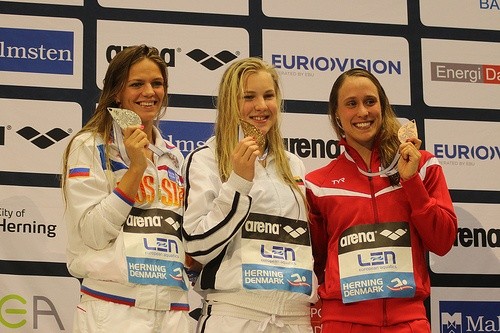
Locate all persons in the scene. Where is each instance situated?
[181,57,319,333]
[60,45,201,333]
[304,68,458,332]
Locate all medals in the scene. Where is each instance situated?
[107,107,142,130]
[398,119,418,145]
[239,118,265,149]
